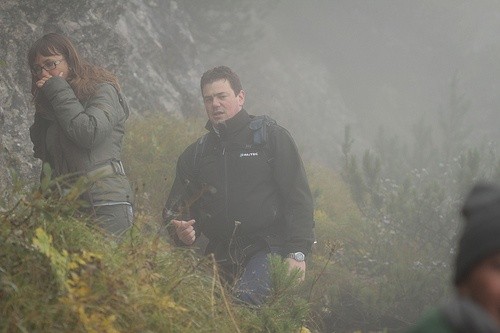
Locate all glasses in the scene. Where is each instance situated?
[31,59,66,76]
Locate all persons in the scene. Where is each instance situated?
[28,34,134,266]
[415,180,500,333]
[162,67,315,308]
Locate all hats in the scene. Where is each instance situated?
[454,182,500,285]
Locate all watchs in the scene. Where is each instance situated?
[287,252,305,262]
